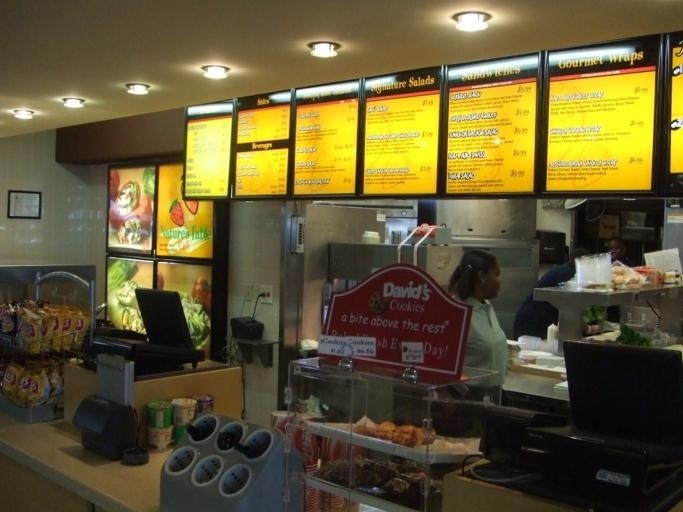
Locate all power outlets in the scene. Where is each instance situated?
[257,285,272,306]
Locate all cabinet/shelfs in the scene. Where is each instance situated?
[303,417,481,512]
[1,295,90,427]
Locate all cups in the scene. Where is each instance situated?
[271,410,364,512]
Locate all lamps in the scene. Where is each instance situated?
[307,41,341,58]
[125,83,150,96]
[14,110,34,120]
[201,65,230,80]
[62,98,85,109]
[451,11,492,33]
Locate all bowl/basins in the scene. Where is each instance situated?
[145,393,218,452]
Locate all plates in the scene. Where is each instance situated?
[621,305,659,330]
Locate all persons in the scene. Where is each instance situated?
[432,249,508,439]
[608,237,627,265]
[513,248,592,342]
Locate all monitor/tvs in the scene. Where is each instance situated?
[562,340,683,441]
[135,288,193,347]
